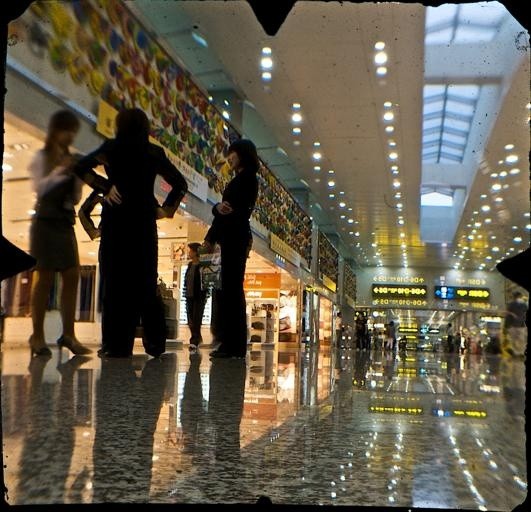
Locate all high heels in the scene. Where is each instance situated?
[56,333,94,357]
[29,334,53,357]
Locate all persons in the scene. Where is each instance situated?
[13,355,92,506]
[355,311,368,351]
[203,138,259,358]
[25,109,93,357]
[183,241,209,351]
[331,349,484,389]
[501,291,528,357]
[73,108,189,359]
[335,312,345,349]
[84,358,178,502]
[178,352,207,454]
[370,321,483,353]
[77,188,113,355]
[503,355,527,431]
[194,358,252,504]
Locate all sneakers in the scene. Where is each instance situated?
[188,343,201,349]
[96,342,168,358]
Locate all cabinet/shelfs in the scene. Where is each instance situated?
[245,298,279,344]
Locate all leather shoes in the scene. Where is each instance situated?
[209,348,247,359]
